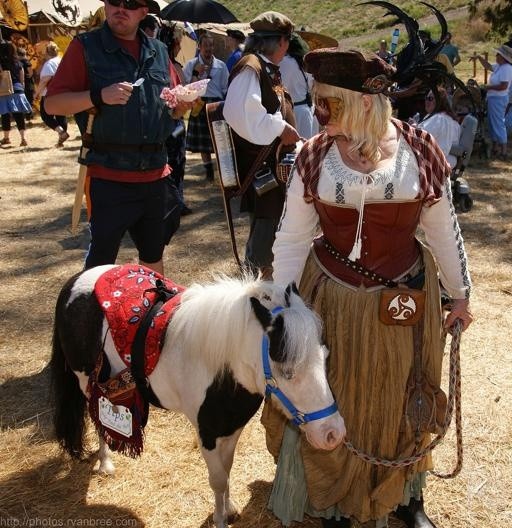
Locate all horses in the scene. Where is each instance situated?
[47,257,347,528]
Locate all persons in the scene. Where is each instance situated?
[33,43,69,146]
[222,10,301,282]
[136,16,327,194]
[267,43,473,527]
[375,32,509,162]
[44,2,194,278]
[2,42,34,146]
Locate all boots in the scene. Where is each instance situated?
[396,497,438,528]
[203,162,213,183]
[321,516,350,528]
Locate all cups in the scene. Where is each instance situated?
[252,168,279,198]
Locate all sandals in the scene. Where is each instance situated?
[0,131,70,146]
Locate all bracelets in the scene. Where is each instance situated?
[192,69,199,77]
[90,88,104,107]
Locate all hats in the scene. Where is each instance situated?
[302,48,397,94]
[493,45,512,64]
[249,11,293,37]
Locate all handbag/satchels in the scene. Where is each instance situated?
[403,385,447,435]
[0,66,15,96]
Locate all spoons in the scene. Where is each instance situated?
[132,76,145,87]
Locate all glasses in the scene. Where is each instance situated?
[109,0,147,10]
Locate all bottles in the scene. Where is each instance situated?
[412,112,419,122]
